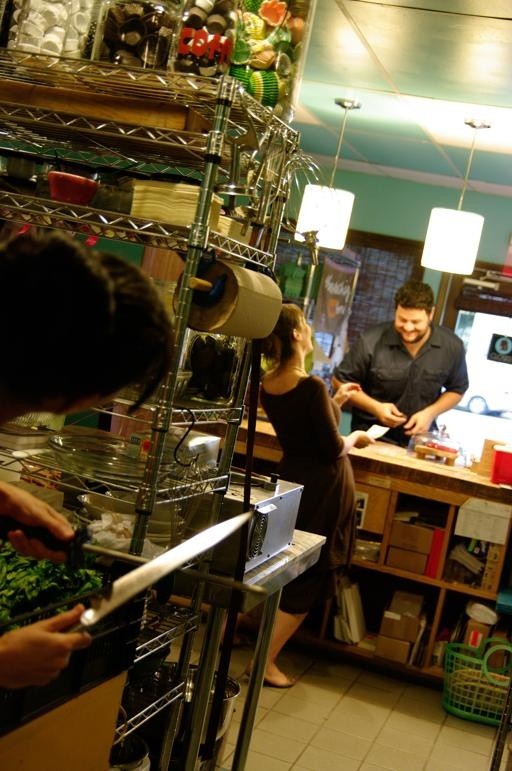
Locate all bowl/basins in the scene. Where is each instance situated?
[45,171,98,209]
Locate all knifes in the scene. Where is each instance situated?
[52,512,255,644]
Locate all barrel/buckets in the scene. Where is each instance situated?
[155,660,242,771]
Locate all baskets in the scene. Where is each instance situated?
[441,638,511,727]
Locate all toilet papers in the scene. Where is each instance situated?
[172,259,284,340]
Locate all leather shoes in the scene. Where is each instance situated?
[245,662,297,688]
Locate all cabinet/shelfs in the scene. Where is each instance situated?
[3,46,303,771]
[303,471,512,698]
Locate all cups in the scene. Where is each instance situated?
[476,439,512,486]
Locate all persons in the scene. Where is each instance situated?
[2,225,175,689]
[219,303,377,688]
[332,281,469,450]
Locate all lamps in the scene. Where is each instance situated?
[291,98,363,250]
[418,121,498,274]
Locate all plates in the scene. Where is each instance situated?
[72,490,185,543]
